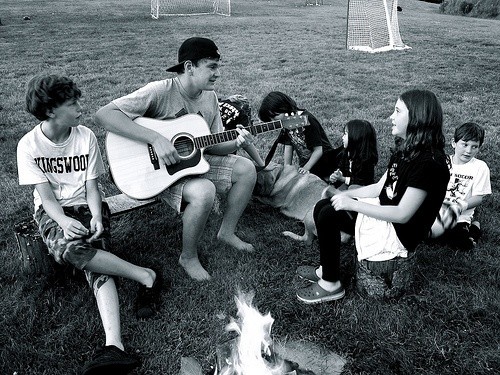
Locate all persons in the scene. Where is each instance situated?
[426,123,492,248]
[216,91,378,244]
[93,37,256,280]
[295,90,453,304]
[16,75,166,375]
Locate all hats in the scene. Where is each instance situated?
[166,36,223,73]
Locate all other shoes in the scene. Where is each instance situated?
[82,345,141,375]
[296,283,346,305]
[134,274,164,320]
[296,265,320,284]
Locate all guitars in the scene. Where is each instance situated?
[105,110,310,201]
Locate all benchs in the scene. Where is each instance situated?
[105,190,162,224]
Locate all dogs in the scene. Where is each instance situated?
[252,160,352,244]
